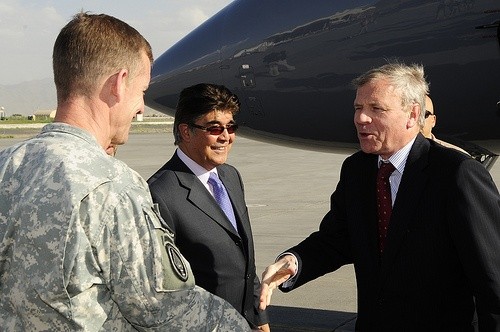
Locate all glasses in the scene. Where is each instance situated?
[425,110,433,119]
[185,121,238,135]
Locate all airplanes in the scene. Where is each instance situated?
[143,0,500,170]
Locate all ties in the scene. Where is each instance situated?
[208,172,238,234]
[377,160,396,255]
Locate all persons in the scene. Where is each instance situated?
[0,11,252,332]
[419,94,473,158]
[146,83,270,332]
[259,62,500,332]
